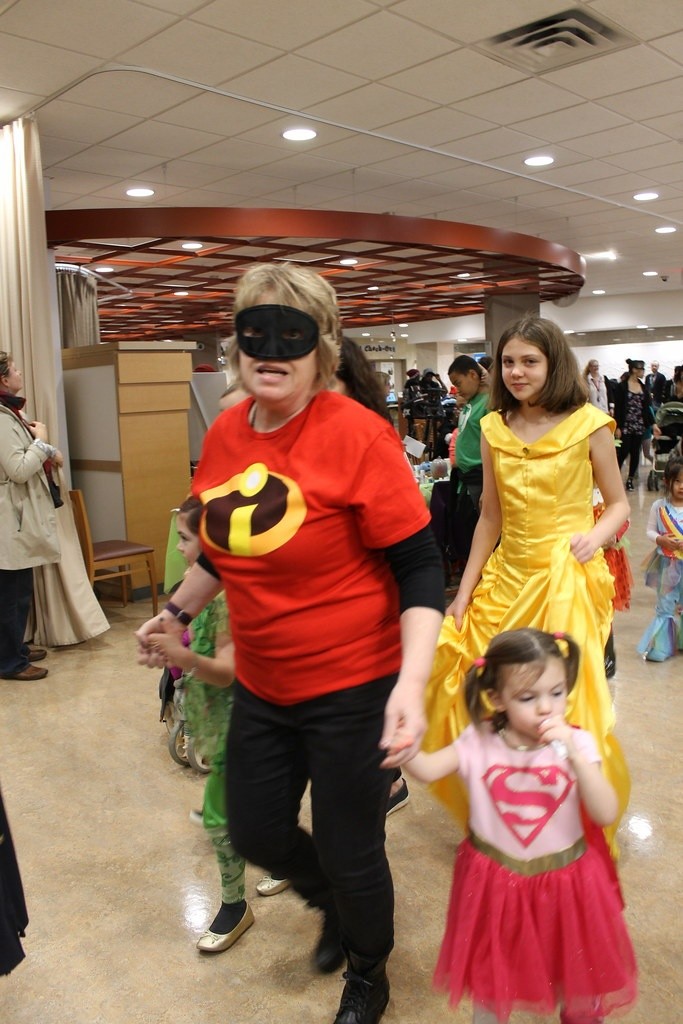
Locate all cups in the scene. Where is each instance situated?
[444,459,452,475]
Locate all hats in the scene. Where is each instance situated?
[407,369,419,379]
[421,368,437,377]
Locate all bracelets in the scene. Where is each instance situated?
[165,602,193,627]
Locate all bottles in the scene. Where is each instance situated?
[418,454,433,484]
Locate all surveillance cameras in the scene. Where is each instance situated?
[661,277,668,281]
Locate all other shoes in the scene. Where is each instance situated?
[316,910,346,973]
[333,960,390,1024]
[385,778,409,816]
[257,873,291,896]
[604,653,616,677]
[196,903,254,952]
[627,477,634,491]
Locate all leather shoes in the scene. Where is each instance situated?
[27,649,47,662]
[0,665,48,680]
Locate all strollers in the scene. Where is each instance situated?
[647,402,683,492]
[432,467,473,586]
[159,663,219,774]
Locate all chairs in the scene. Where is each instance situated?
[67,490,157,619]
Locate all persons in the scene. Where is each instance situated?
[592,476,631,679]
[582,358,614,416]
[641,361,683,464]
[402,369,448,405]
[0,350,62,681]
[388,627,641,1024]
[421,318,633,857]
[142,496,291,952]
[331,336,409,814]
[637,455,683,661]
[611,358,662,491]
[134,264,447,1024]
[447,354,494,515]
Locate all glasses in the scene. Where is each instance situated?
[637,367,645,370]
